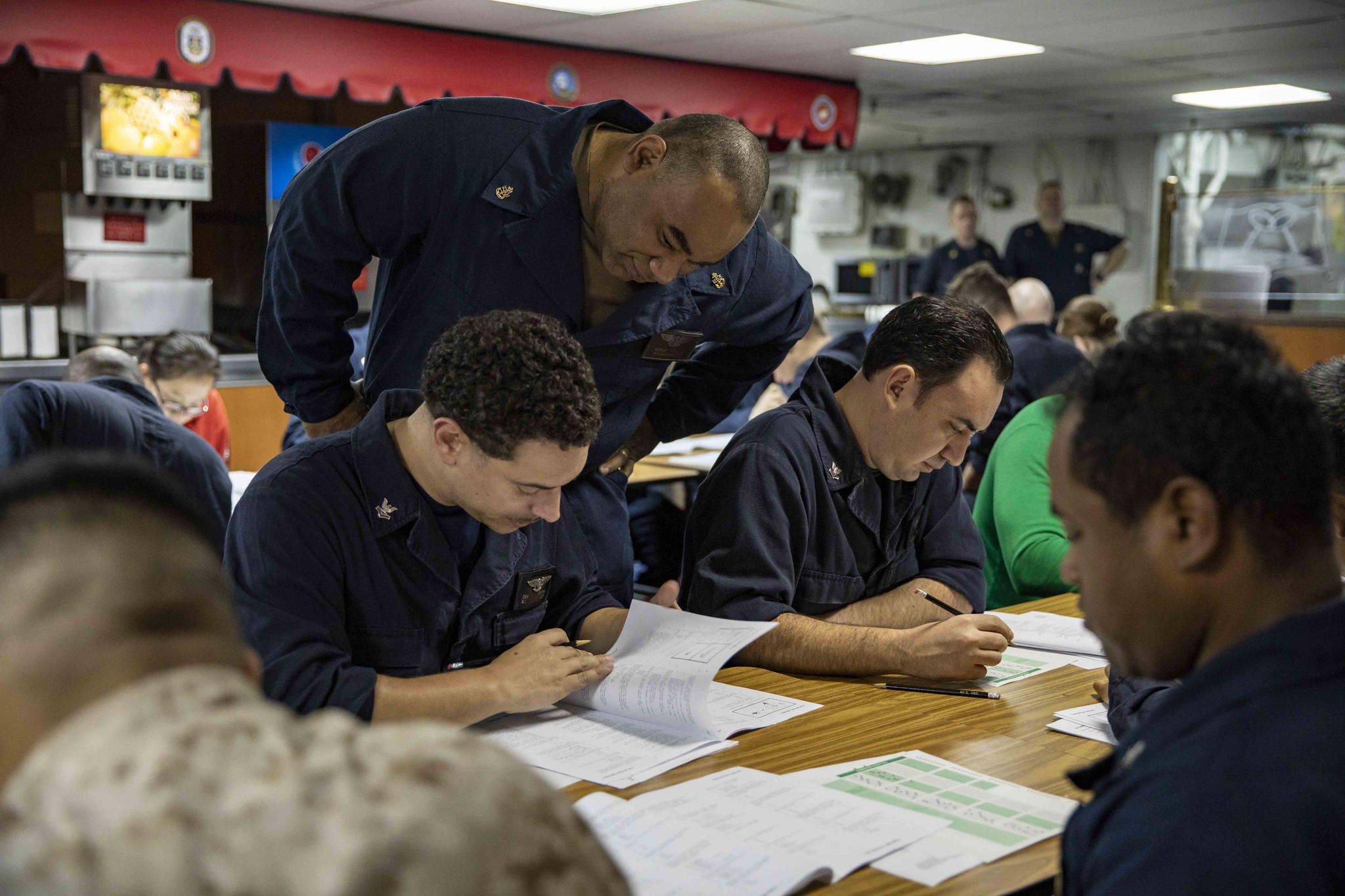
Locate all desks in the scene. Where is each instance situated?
[626,431,736,508]
[452,591,1119,896]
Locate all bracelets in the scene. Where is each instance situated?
[1096,273,1103,281]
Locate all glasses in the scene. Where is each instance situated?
[148,371,208,416]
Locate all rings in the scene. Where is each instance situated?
[621,450,628,458]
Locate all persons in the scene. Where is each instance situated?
[623,264,1121,612]
[1004,178,1127,314]
[0,447,629,896]
[255,99,814,501]
[281,309,371,453]
[911,194,1003,299]
[676,293,1013,682]
[222,311,636,738]
[0,331,234,530]
[1040,306,1345,894]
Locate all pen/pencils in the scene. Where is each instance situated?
[448,640,594,668]
[914,588,1016,648]
[871,683,1001,699]
